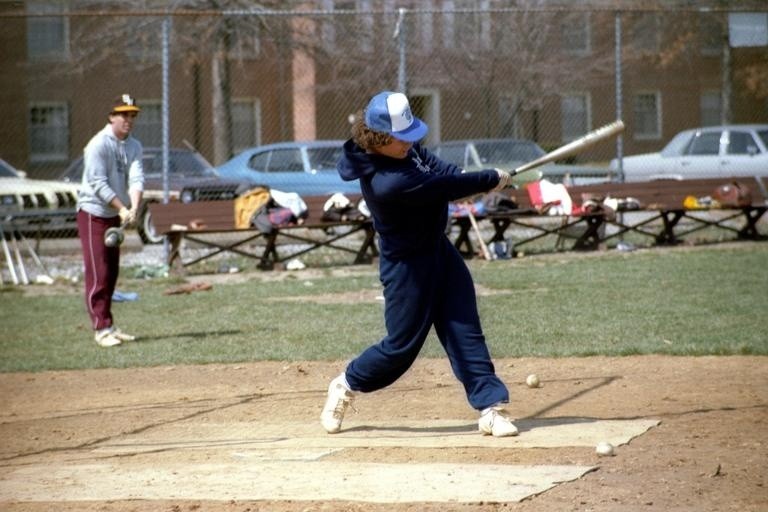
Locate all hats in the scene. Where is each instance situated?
[108,93,143,116]
[363,91,429,143]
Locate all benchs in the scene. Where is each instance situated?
[145,176,767,277]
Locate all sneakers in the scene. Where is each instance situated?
[322,373,356,435]
[476,405,520,439]
[93,323,136,349]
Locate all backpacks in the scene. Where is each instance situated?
[230,183,308,231]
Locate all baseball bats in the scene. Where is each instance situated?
[104,220,128,248]
[507,119,626,177]
[464,201,492,261]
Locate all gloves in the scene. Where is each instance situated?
[118,208,134,224]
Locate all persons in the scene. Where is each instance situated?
[319,91,519,437]
[76,94,145,347]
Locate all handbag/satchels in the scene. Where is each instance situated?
[481,193,519,215]
[603,197,642,213]
[581,198,604,214]
[684,195,720,209]
[714,180,751,209]
[524,180,580,216]
[448,200,486,218]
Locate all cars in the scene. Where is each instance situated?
[0,158,81,234]
[425,138,622,187]
[608,124,768,183]
[58,146,254,245]
[213,140,364,195]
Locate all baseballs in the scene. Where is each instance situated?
[527,374,539,387]
[596,443,613,457]
